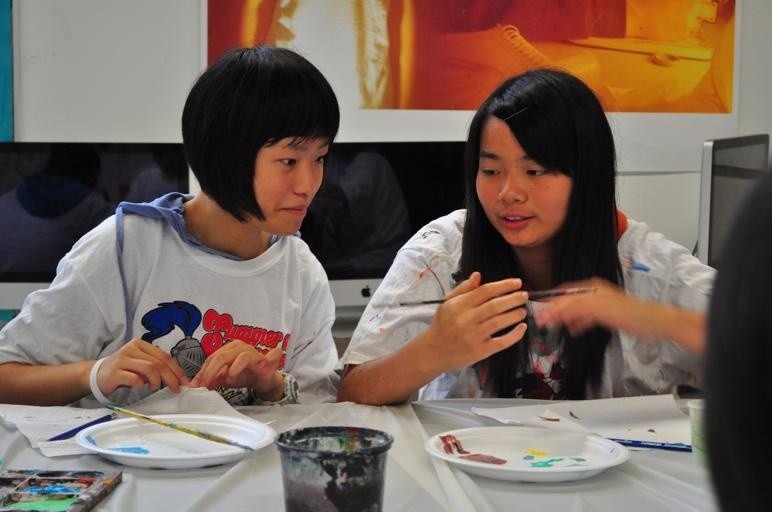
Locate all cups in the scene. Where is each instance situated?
[274,428,393,511]
[687,398,711,484]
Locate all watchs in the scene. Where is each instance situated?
[251,369,299,405]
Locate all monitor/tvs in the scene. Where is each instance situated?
[1,140,191,312]
[299,139,475,311]
[697,131,772,271]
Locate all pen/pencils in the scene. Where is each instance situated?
[46,412,118,442]
[608,438,692,453]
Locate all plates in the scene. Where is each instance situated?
[425,425,632,484]
[77,414,278,467]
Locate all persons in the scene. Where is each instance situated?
[337,66,719,406]
[0,43,341,408]
[0,141,114,286]
[310,129,416,283]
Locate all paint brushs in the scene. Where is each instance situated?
[371,286,599,309]
[102,403,255,452]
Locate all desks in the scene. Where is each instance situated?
[0,395,720,512]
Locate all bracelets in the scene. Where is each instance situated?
[87,354,132,407]
